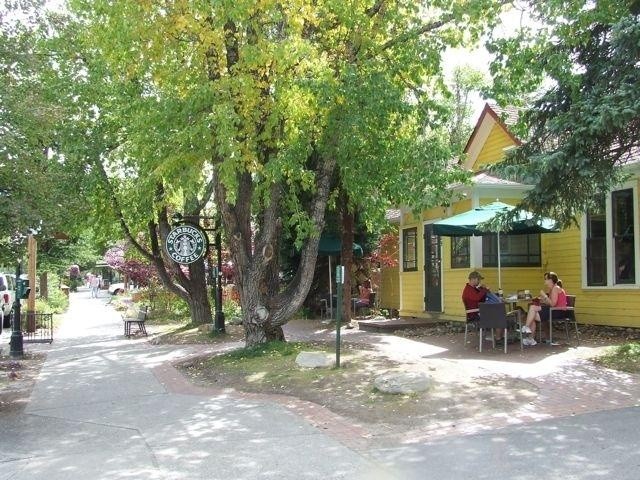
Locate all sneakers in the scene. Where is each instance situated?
[494,325,537,350]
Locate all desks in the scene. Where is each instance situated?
[485,293,540,313]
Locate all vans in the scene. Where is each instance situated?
[0,269,31,337]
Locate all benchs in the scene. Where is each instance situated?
[119,304,151,338]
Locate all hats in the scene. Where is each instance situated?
[469,271,485,278]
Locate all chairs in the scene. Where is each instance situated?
[477,302,525,353]
[538,294,582,347]
[321,290,377,322]
[463,297,498,349]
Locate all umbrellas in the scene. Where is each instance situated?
[434,196,562,297]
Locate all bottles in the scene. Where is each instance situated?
[498,288,503,299]
[524,289,531,300]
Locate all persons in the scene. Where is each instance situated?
[516,271,568,348]
[84,270,105,297]
[294,232,364,295]
[462,270,507,346]
[353,280,372,314]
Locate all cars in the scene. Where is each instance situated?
[108,280,138,295]
